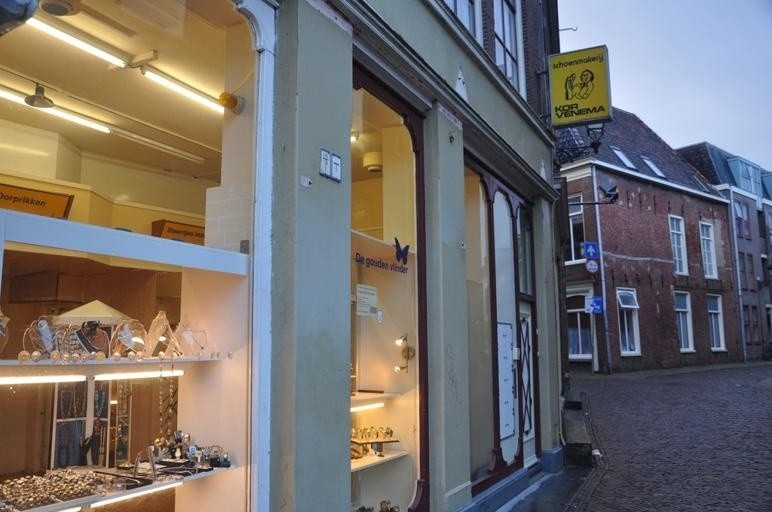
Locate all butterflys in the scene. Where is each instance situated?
[394,236,410,266]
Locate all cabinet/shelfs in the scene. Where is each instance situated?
[0,355,233,512]
[350,391,408,473]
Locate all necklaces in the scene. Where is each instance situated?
[163,466,200,477]
[82,321,223,352]
[117,475,142,487]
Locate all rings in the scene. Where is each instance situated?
[0,473,99,509]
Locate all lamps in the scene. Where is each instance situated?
[568,184,619,206]
[552,123,603,172]
[394,332,415,374]
[24,86,54,111]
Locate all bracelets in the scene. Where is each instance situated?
[348,421,396,460]
